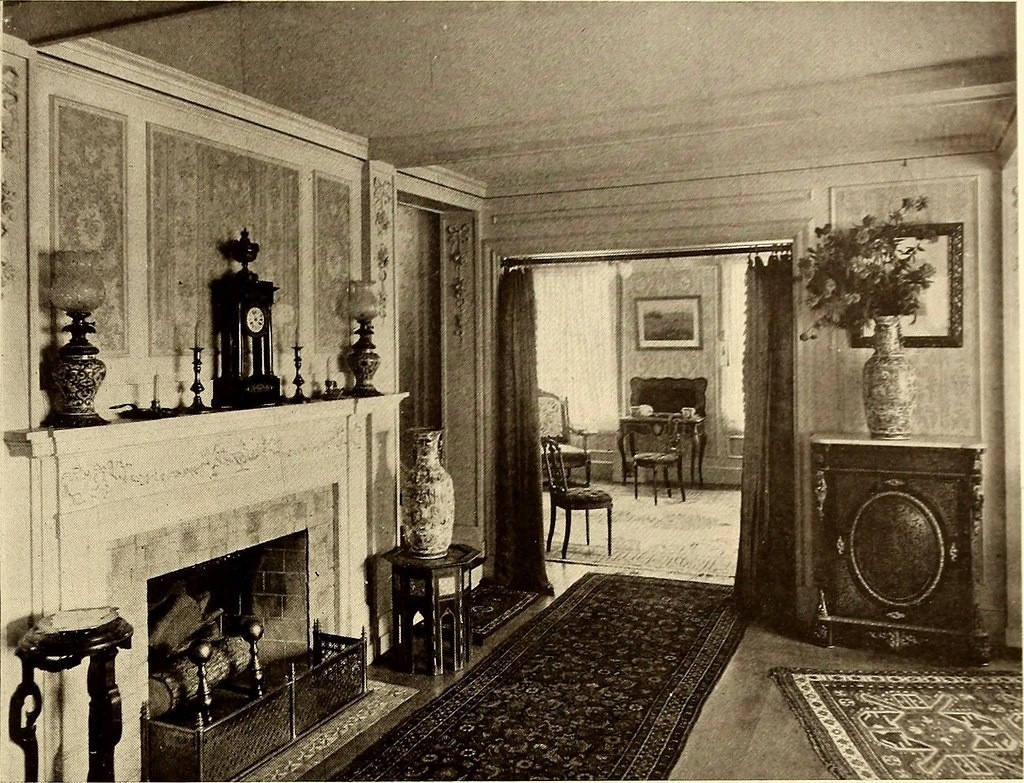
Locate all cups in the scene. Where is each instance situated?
[681,408,695,419]
[631,407,641,416]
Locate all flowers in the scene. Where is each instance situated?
[798,195,938,341]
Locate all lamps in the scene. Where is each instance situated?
[348,280,380,349]
[48,251,107,356]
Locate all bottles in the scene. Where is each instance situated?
[402,424,456,560]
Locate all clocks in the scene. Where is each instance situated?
[212,227,283,409]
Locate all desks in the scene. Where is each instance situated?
[617,412,708,489]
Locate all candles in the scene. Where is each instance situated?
[295,328,299,346]
[153,370,160,401]
[195,320,204,347]
[327,358,331,381]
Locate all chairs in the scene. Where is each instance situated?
[633,413,686,506]
[540,436,613,559]
[538,388,597,487]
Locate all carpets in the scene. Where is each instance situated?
[768,666,1023,781]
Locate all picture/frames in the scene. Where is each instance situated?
[633,295,704,351]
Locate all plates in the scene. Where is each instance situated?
[654,412,681,417]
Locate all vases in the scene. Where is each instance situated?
[347,347,384,398]
[42,354,111,427]
[402,428,455,560]
[862,316,918,441]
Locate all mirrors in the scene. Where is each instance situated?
[850,222,964,349]
